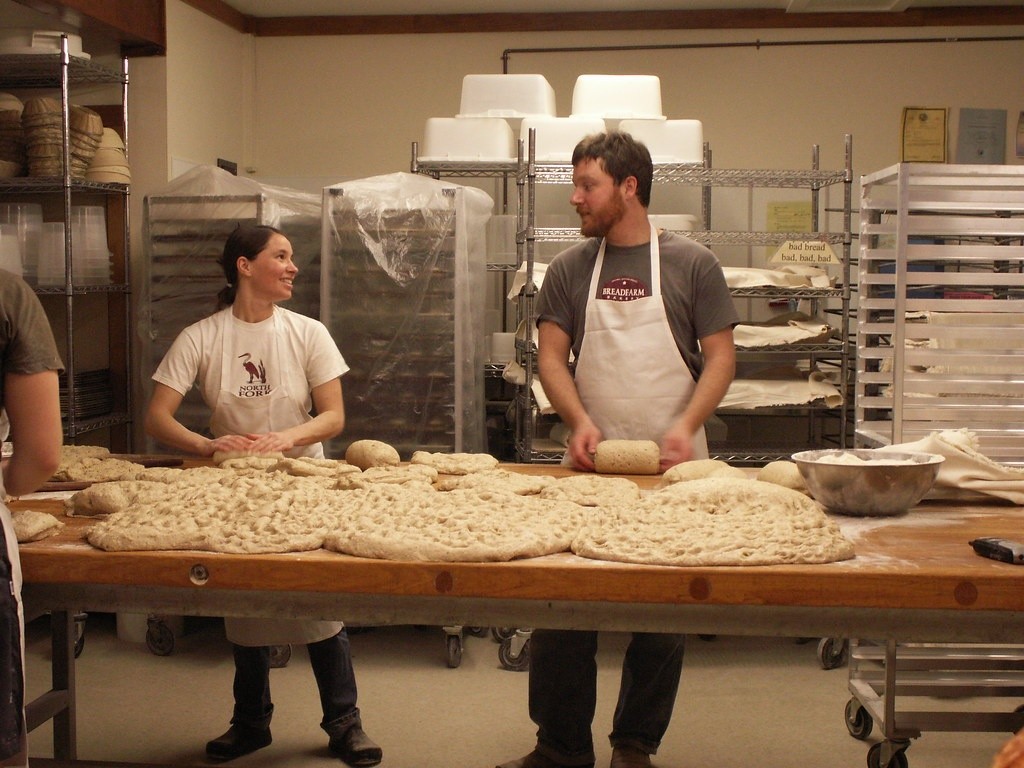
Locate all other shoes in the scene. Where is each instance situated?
[327,726,382,766]
[206,724,272,762]
[608,744,650,768]
[495,748,561,767]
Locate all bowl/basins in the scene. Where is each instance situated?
[790,448,946,515]
[86,127,131,186]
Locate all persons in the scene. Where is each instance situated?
[0,262,68,768]
[490,131,740,768]
[148,223,386,768]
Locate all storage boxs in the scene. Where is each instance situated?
[648,214,703,232]
[570,74,668,120]
[516,118,609,161]
[455,74,557,118]
[492,333,517,362]
[0,202,115,287]
[618,119,704,163]
[0,28,92,60]
[417,117,518,162]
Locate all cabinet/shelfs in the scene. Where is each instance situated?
[843,161,1023,767]
[140,186,488,669]
[0,35,135,659]
[409,129,1023,669]
